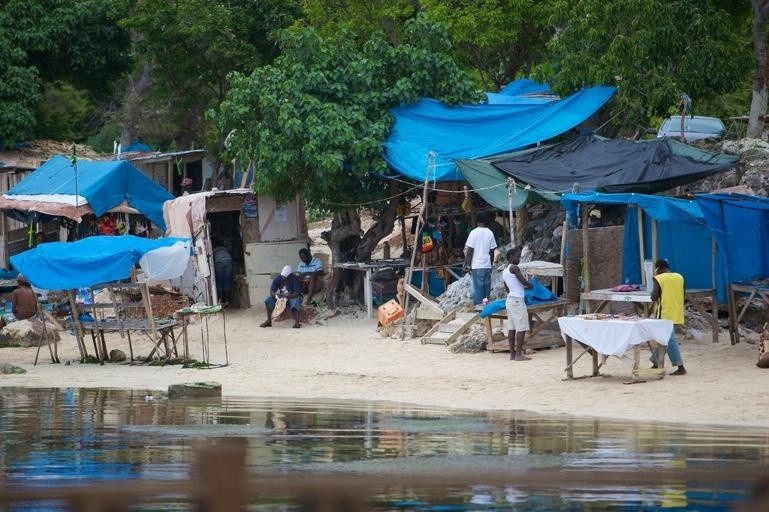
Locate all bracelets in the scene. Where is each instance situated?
[467,266,470,269]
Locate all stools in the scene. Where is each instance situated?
[180,302,230,371]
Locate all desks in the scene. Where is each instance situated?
[517,260,566,297]
[481,296,571,354]
[557,313,675,383]
[579,286,720,344]
[727,280,768,346]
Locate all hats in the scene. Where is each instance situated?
[281,266,292,278]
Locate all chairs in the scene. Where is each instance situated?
[307,251,334,306]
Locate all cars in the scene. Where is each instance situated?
[655,115,727,144]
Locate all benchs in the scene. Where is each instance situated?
[66,282,184,362]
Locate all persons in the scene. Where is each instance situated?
[259,264,305,330]
[294,247,325,306]
[212,243,235,306]
[38,231,46,242]
[461,210,497,308]
[11,273,38,320]
[180,177,193,196]
[501,249,534,361]
[647,258,687,376]
